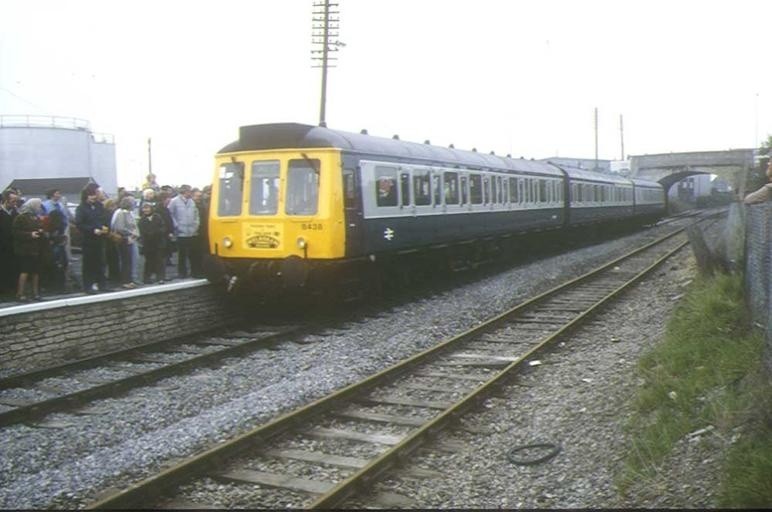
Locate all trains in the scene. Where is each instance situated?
[201,121,667,302]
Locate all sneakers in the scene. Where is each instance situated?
[14,294,44,303]
[121,282,136,289]
[85,286,113,295]
[158,278,171,284]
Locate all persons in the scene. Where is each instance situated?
[0,173,212,303]
[744,157,772,205]
